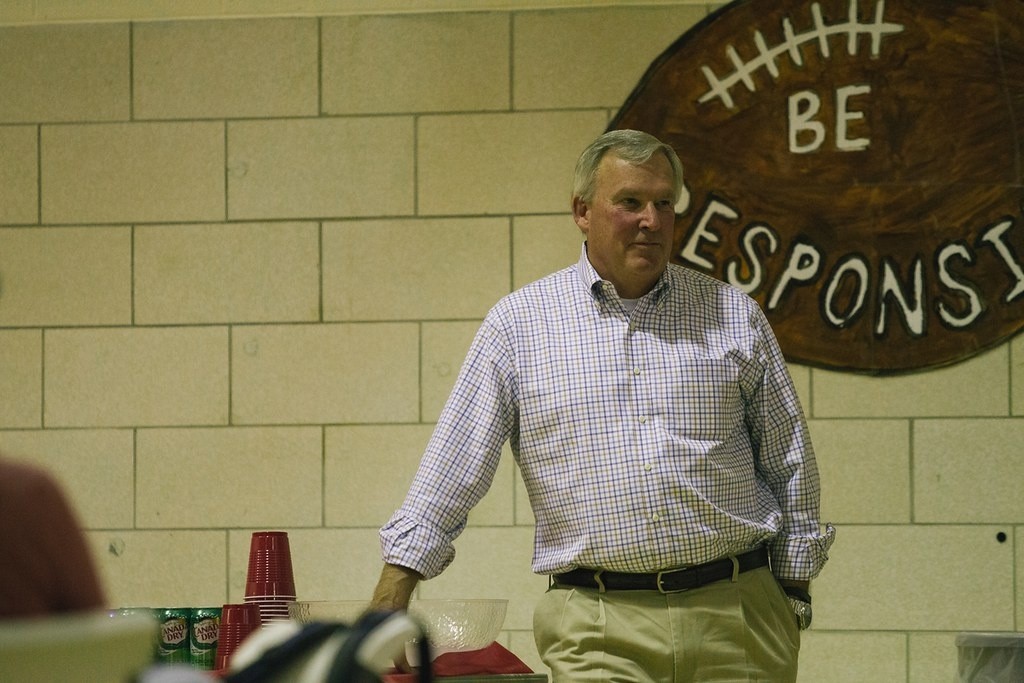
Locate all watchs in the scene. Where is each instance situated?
[789,598,813,631]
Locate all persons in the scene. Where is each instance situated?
[0,457,157,683]
[362,128,837,683]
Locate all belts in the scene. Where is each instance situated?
[552,547,768,594]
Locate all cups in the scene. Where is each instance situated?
[212,530,297,673]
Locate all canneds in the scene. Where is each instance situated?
[110,607,222,672]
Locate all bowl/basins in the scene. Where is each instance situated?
[287,598,511,675]
